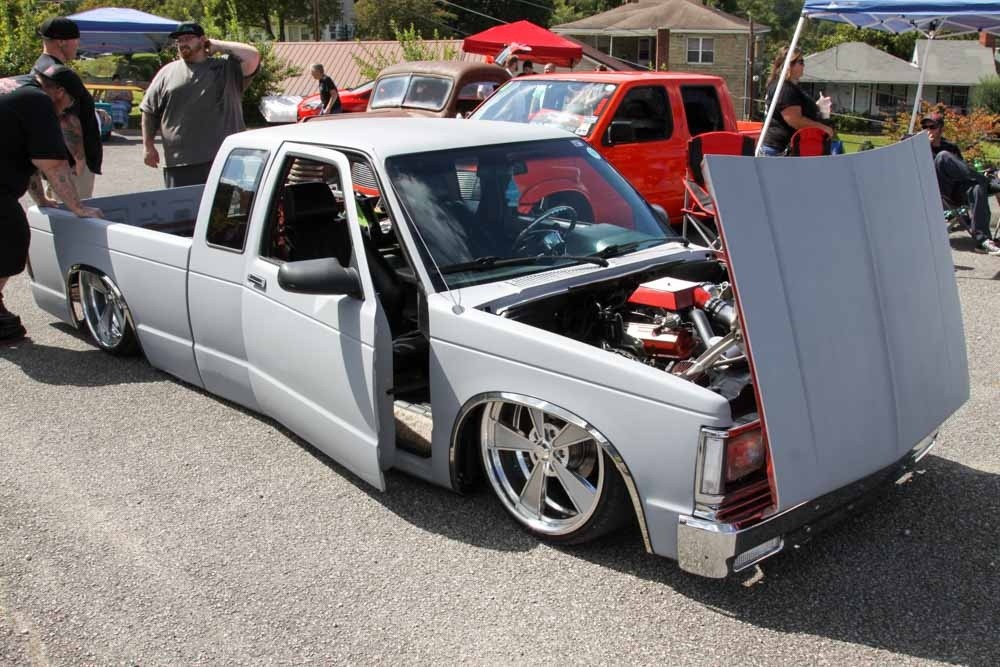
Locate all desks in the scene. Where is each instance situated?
[83,83,144,103]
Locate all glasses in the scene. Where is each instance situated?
[922,125,938,130]
[795,59,806,64]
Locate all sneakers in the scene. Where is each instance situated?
[975,239,1000,255]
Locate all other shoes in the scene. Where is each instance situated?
[1,294,27,340]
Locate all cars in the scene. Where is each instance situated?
[295,76,478,122]
[287,57,518,188]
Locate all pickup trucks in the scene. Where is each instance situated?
[20,107,974,590]
[352,70,765,228]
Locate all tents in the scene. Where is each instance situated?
[463,20,583,68]
[65,7,182,66]
[754,0,1000,158]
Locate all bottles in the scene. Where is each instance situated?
[974,159,993,171]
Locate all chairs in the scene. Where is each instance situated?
[680,131,756,250]
[785,127,832,157]
[285,182,430,389]
[903,132,973,235]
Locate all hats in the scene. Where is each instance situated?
[33,64,85,103]
[920,112,944,125]
[40,17,81,41]
[168,23,205,36]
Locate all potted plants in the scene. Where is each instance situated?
[242,36,306,124]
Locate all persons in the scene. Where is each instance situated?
[139,23,261,188]
[592,64,606,71]
[544,63,555,73]
[29,18,103,204]
[503,54,518,77]
[517,60,538,77]
[0,65,104,340]
[311,64,342,115]
[901,114,1000,256]
[759,46,834,156]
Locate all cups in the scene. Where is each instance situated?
[816,97,831,119]
